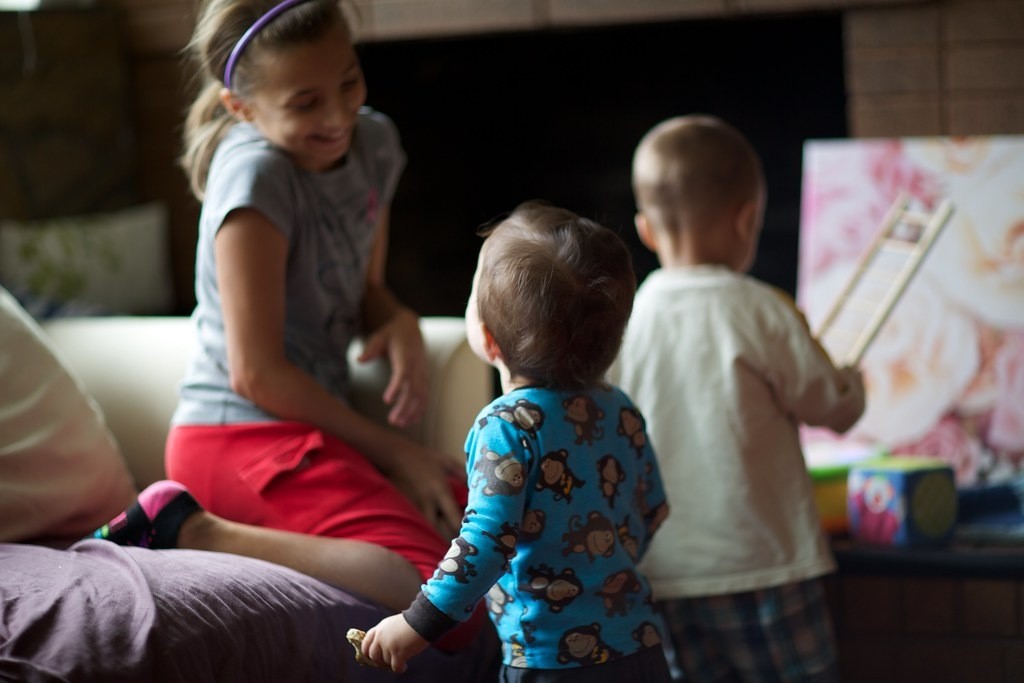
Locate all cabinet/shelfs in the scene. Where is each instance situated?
[831,534,1024,682]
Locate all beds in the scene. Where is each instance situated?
[0,311,491,683]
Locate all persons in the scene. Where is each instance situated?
[79,0,470,613]
[360,201,676,683]
[600,116,867,683]
